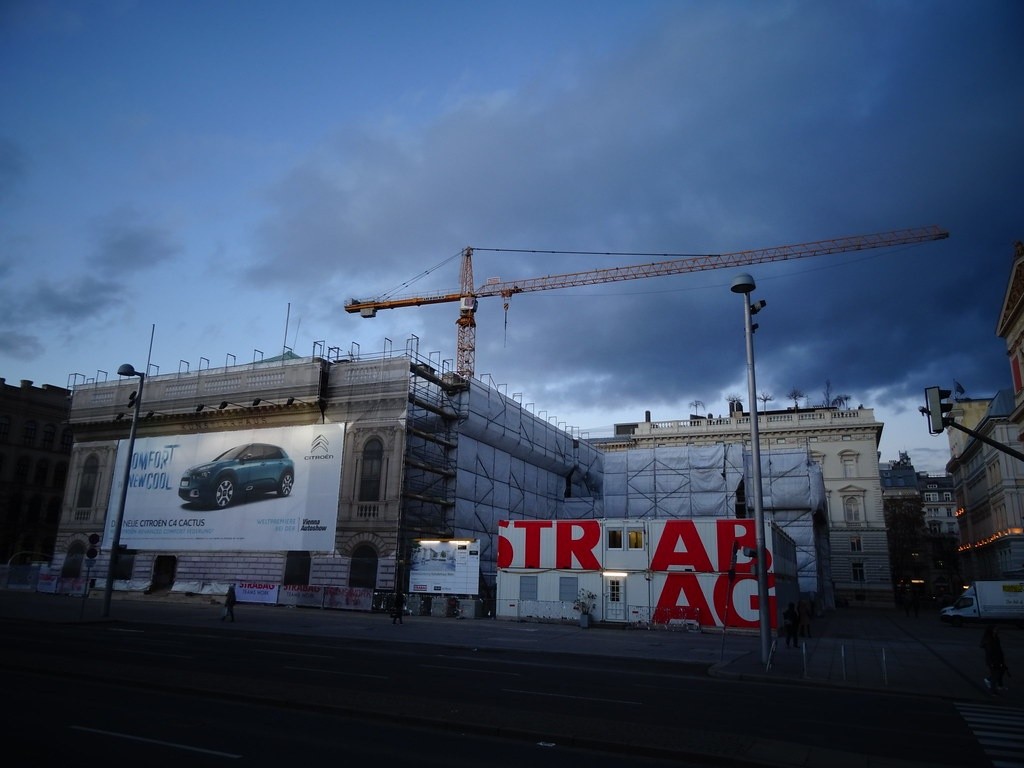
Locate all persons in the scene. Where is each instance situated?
[782,602,800,649]
[902,589,920,617]
[392,588,406,625]
[797,598,812,638]
[981,624,1008,691]
[221,586,236,623]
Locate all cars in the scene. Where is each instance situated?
[178,443,295,509]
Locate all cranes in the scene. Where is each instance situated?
[344,224,950,379]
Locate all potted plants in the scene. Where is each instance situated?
[573,588,597,629]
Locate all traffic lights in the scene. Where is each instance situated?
[938,387,957,429]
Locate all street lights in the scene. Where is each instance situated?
[724,267,776,675]
[100,362,147,621]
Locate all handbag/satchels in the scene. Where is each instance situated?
[221,607,228,616]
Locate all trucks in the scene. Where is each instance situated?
[940,579,1024,627]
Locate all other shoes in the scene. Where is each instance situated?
[984,678,991,689]
[997,686,1008,691]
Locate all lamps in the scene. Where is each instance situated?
[286,396,312,406]
[144,410,167,419]
[219,400,246,409]
[116,412,143,421]
[253,398,278,407]
[196,404,218,412]
